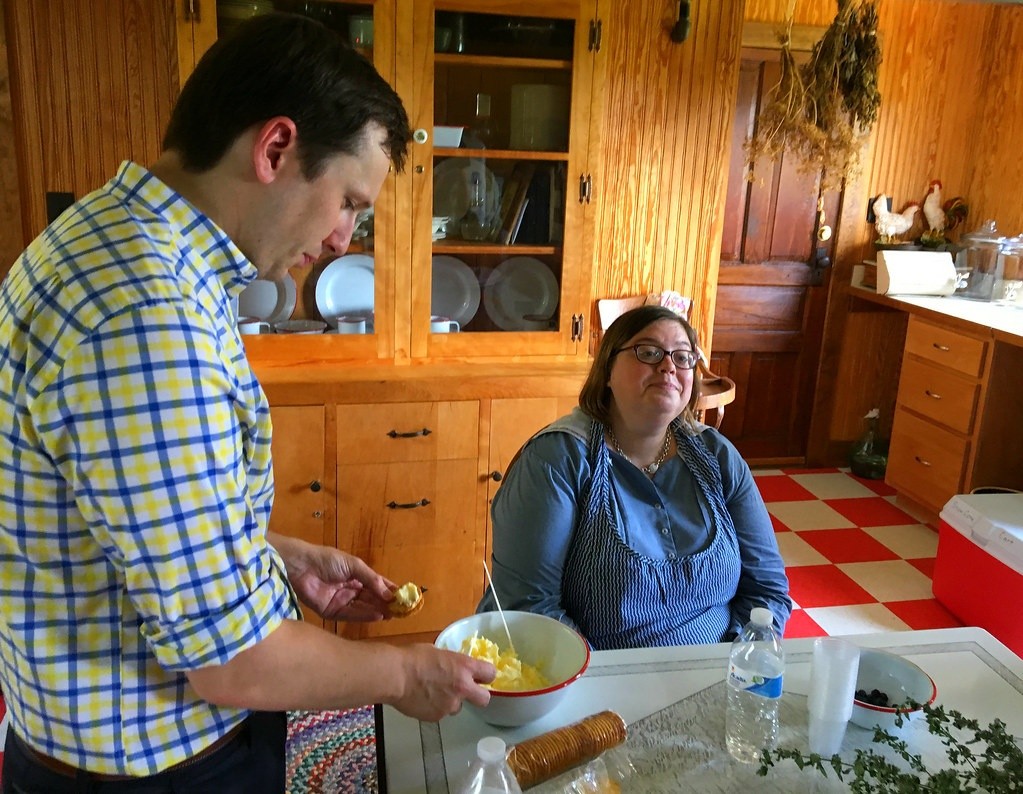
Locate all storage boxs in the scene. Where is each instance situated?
[932,492,1023,660]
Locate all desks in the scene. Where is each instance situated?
[374,626,1023,792]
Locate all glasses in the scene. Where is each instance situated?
[616,344,701,369]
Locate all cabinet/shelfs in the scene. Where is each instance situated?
[882,311,1023,531]
[175,0,596,647]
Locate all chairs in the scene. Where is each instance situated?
[599,294,737,432]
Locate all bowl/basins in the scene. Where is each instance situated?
[873,238,952,251]
[274,320,327,334]
[850,647,937,730]
[434,610,590,730]
[434,126,464,148]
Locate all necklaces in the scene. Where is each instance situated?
[605,422,673,475]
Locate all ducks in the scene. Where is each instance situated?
[848,407,888,480]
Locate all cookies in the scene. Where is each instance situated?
[506,710,627,790]
[388,585,424,619]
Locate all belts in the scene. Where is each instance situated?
[14,717,244,782]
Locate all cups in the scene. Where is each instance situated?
[238,316,271,334]
[452,115,500,149]
[430,315,460,333]
[431,217,450,241]
[808,637,860,759]
[298,1,336,30]
[349,15,374,48]
[509,84,568,152]
[435,28,452,51]
[336,317,365,332]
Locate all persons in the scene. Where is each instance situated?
[0,12,496,794]
[474,305,792,652]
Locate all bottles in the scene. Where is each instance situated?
[584,679,729,793]
[521,161,551,245]
[450,14,466,53]
[216,0,277,41]
[848,408,889,480]
[460,736,522,794]
[954,219,1023,302]
[726,609,786,764]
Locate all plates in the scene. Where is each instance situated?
[238,270,298,331]
[483,257,559,331]
[434,158,499,235]
[316,254,375,328]
[432,256,481,329]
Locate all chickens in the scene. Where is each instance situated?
[923,180,968,237]
[872,192,921,243]
